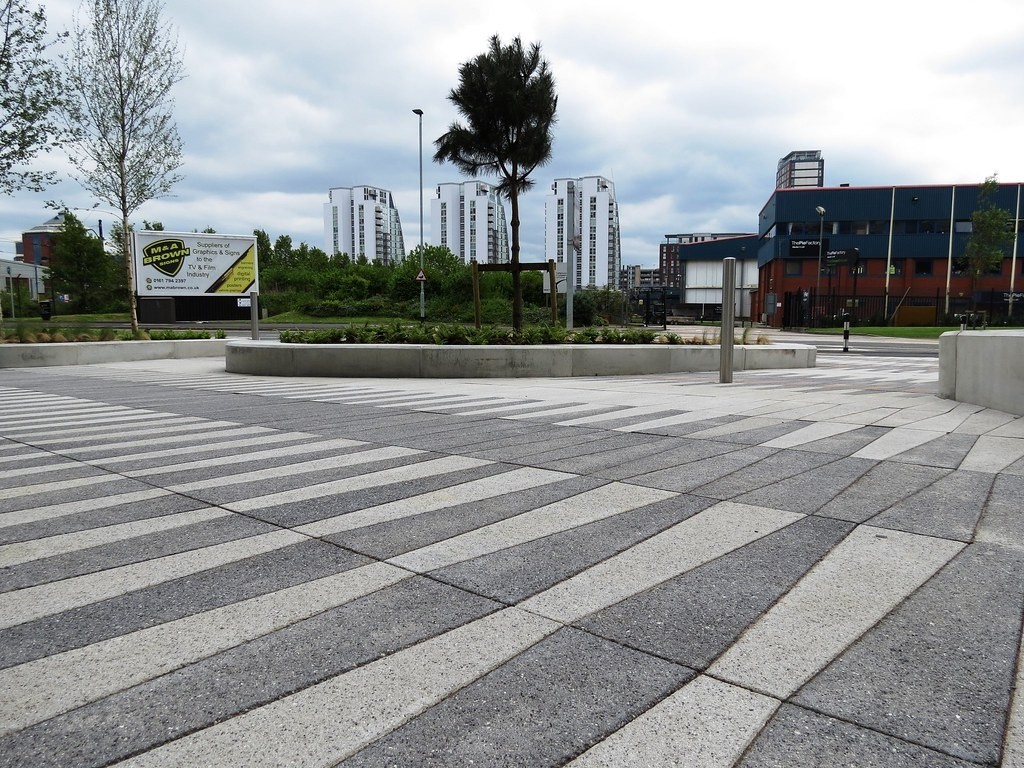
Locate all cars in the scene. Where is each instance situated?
[642,312,673,325]
[628,314,644,322]
[959,313,992,326]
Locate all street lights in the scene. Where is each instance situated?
[851,248,859,310]
[41,257,56,316]
[412,109,426,324]
[7,266,15,319]
[17,274,22,318]
[82,219,106,248]
[815,205,826,298]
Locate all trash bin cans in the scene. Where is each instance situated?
[39,302,52,320]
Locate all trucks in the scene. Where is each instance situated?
[657,312,695,325]
